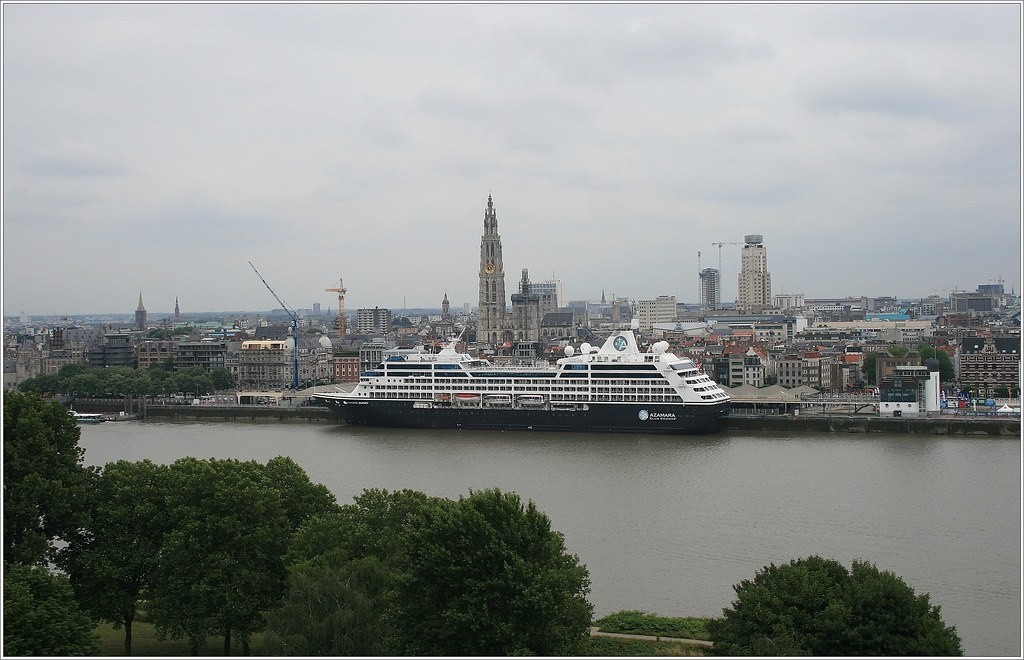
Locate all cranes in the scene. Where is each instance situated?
[713,242,741,275]
[324,278,349,346]
[248,261,300,392]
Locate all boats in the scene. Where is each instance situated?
[454,393,480,404]
[482,395,512,406]
[433,394,451,403]
[66,403,106,423]
[517,394,545,406]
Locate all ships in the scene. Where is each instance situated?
[311,327,732,436]
